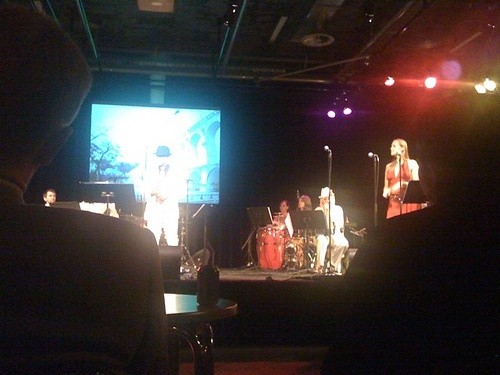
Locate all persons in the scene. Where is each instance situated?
[43,188,56,207]
[0,0,170,375]
[383,139,423,219]
[140,146,179,246]
[322,91,500,375]
[274,187,348,273]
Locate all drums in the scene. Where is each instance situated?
[257,229,286,269]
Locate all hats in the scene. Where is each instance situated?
[154,145,171,157]
[319,187,333,198]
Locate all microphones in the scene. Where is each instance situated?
[367,152,379,159]
[324,145,332,154]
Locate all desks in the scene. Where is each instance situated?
[164,293,242,375]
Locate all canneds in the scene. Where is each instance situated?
[196,264,220,305]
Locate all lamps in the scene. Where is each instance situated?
[328,83,354,119]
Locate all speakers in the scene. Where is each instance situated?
[159,246,183,279]
[191,248,209,269]
